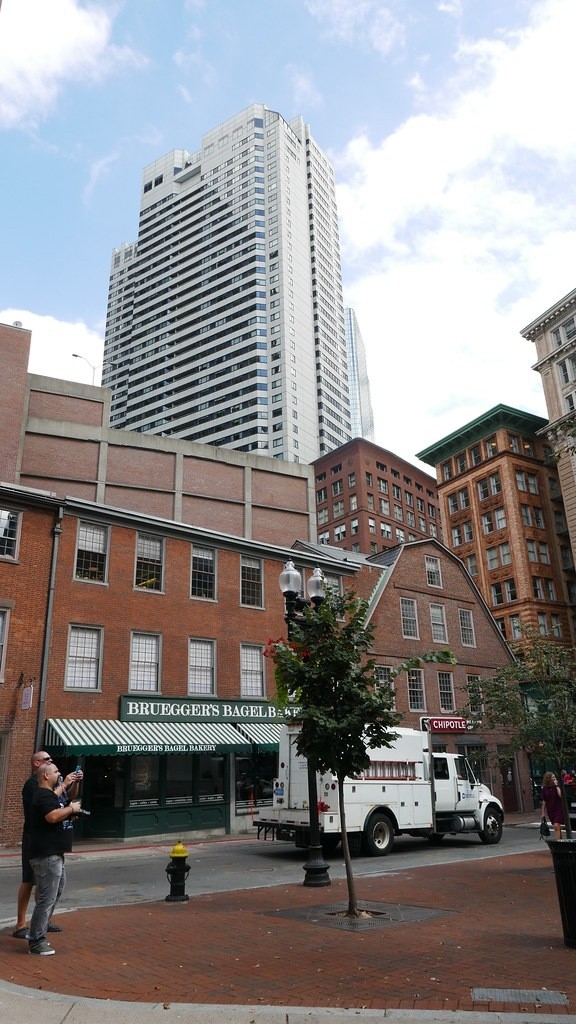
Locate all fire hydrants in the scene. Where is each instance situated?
[163,840,192,901]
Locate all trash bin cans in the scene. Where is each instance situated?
[543,838,576,950]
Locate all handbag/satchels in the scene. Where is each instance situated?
[539,823,551,836]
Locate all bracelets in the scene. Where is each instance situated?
[69,805,75,814]
[555,785,560,787]
[60,784,66,789]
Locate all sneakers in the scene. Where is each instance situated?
[30,935,56,956]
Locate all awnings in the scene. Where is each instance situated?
[235,722,283,752]
[43,718,253,760]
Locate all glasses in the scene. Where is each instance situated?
[38,756,53,761]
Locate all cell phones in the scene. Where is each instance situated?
[76,765,81,782]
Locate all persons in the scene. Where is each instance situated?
[9,750,83,941]
[539,772,568,840]
[560,770,576,784]
[235,772,265,799]
[24,762,82,957]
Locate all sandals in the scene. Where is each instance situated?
[47,922,62,933]
[13,925,30,938]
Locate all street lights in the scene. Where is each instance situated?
[278,555,333,887]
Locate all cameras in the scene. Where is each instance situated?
[72,799,90,816]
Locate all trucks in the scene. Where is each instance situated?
[252,719,505,858]
